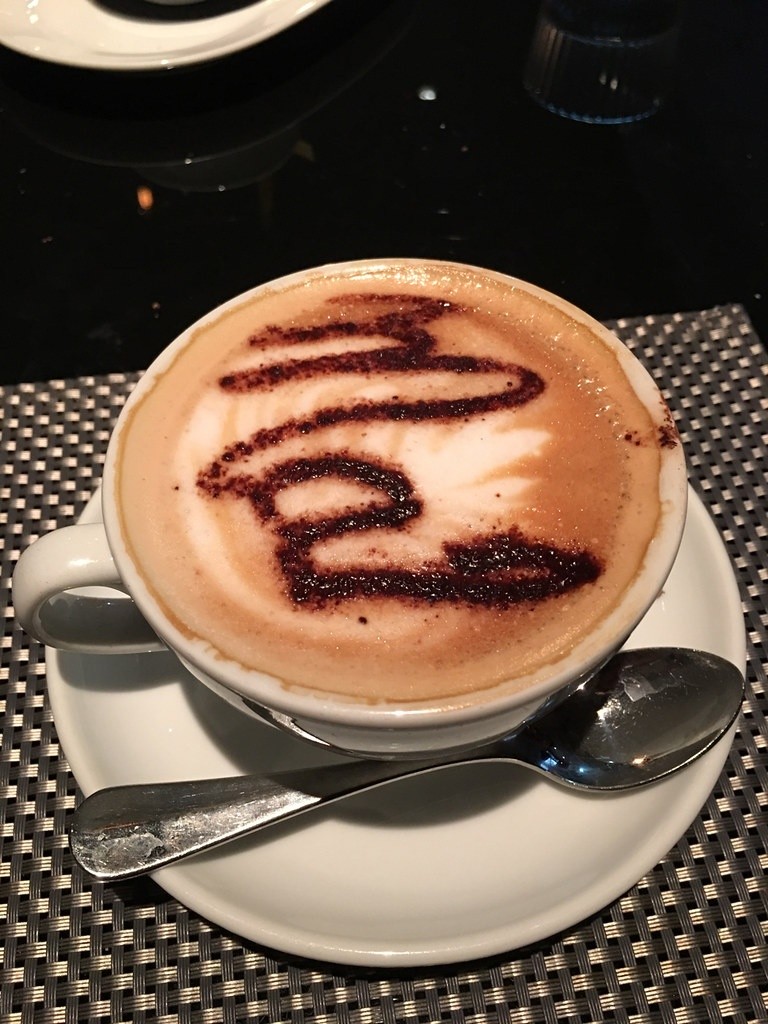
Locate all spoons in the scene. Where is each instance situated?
[67,646,747,884]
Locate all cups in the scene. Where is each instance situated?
[521,0,682,47]
[11,257,689,762]
[519,14,668,125]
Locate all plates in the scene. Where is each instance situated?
[12,479,747,969]
[0,0,331,72]
